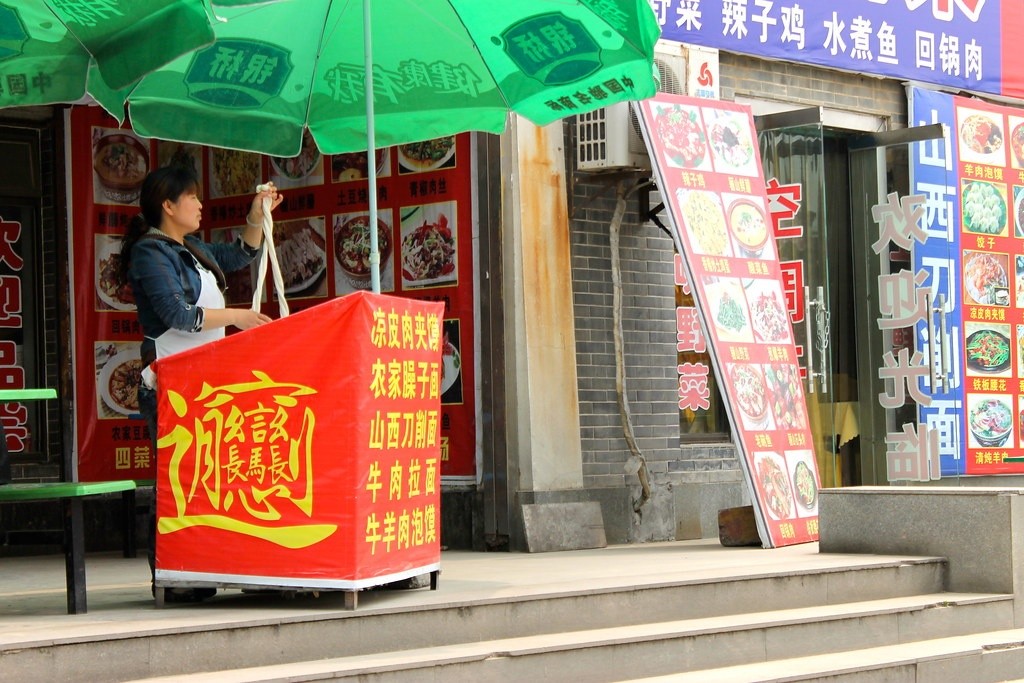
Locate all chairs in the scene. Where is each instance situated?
[0,388,137,614]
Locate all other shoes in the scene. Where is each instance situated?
[152,583,216,603]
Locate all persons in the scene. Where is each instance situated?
[116,166,284,605]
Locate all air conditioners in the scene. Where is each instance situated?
[576,37,720,172]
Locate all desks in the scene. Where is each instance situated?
[821,402,859,485]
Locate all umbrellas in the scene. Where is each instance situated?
[0,0,664,296]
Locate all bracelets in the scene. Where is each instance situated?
[246,214,263,228]
